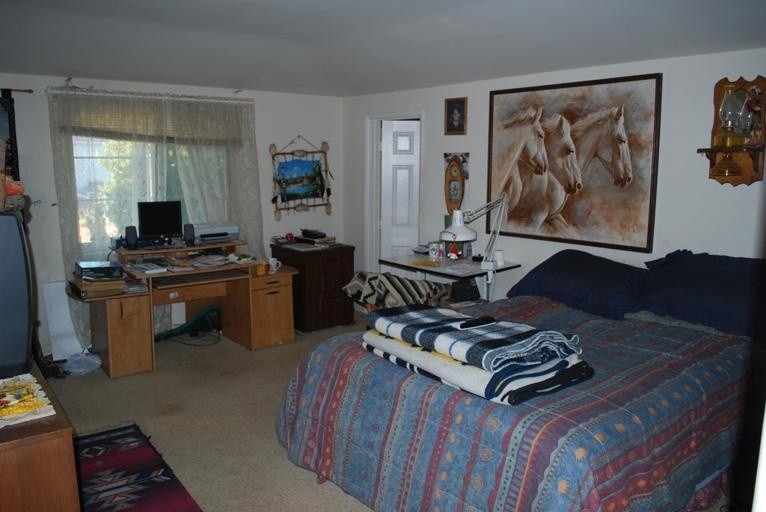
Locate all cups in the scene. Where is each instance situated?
[494,249,505,266]
[267,258,282,275]
[429,243,441,258]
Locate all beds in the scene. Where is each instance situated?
[274,248,765,511]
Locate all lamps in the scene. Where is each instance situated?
[694,72,765,189]
[439,192,512,284]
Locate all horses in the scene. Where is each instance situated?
[490,103,633,234]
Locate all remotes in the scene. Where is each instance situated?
[460,316,495,328]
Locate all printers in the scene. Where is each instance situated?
[194,224,240,246]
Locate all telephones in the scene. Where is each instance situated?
[301,229,326,239]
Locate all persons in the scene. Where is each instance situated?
[447,107,464,132]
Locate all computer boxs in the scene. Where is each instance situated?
[75,260,123,279]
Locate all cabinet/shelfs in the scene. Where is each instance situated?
[268,236,358,335]
[0,362,80,511]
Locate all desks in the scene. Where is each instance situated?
[377,242,521,306]
[67,236,300,380]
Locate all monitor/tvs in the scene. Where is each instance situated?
[137,202,182,248]
[0,211,37,378]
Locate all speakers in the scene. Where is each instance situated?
[125,226,137,250]
[184,224,194,246]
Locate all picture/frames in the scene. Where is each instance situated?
[442,96,468,136]
[484,72,664,254]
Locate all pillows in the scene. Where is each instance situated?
[644,244,764,343]
[503,249,662,326]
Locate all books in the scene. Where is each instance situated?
[128,249,258,273]
[284,231,342,252]
[0,372,56,427]
[67,259,148,298]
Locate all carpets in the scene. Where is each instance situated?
[72,419,206,512]
